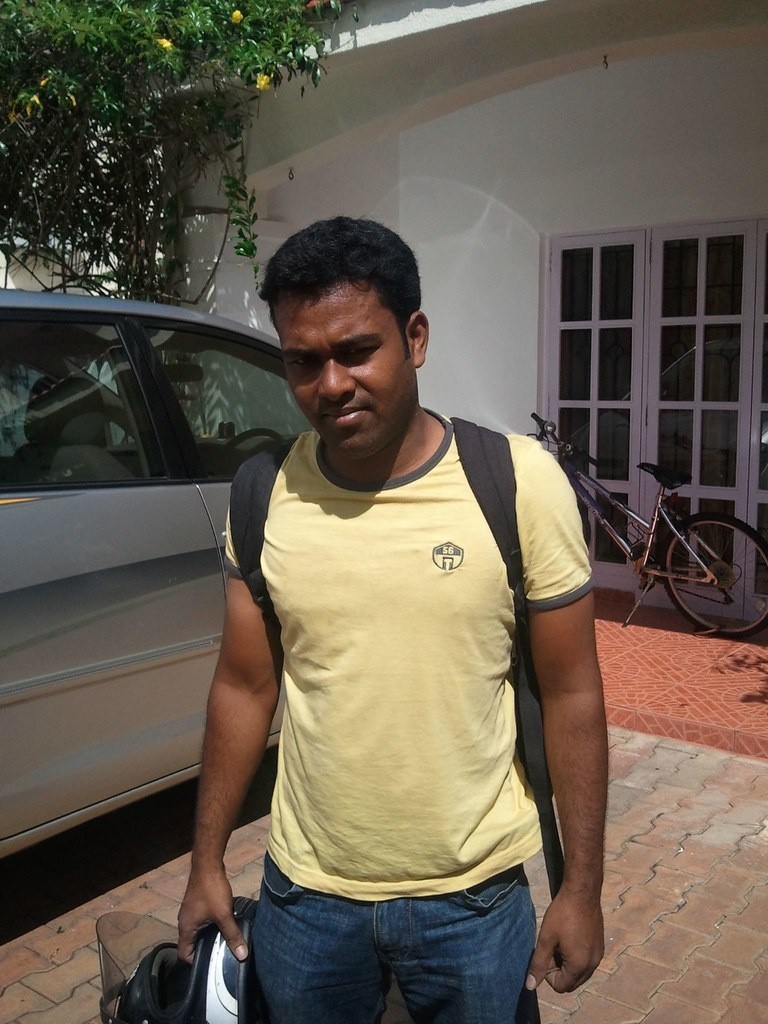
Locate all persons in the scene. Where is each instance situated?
[176,218,608,1024]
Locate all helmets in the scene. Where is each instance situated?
[115,896,259,1024]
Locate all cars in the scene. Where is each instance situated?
[0,289,283,865]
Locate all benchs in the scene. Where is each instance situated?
[0,367,132,483]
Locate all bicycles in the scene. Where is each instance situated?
[524,410,768,639]
